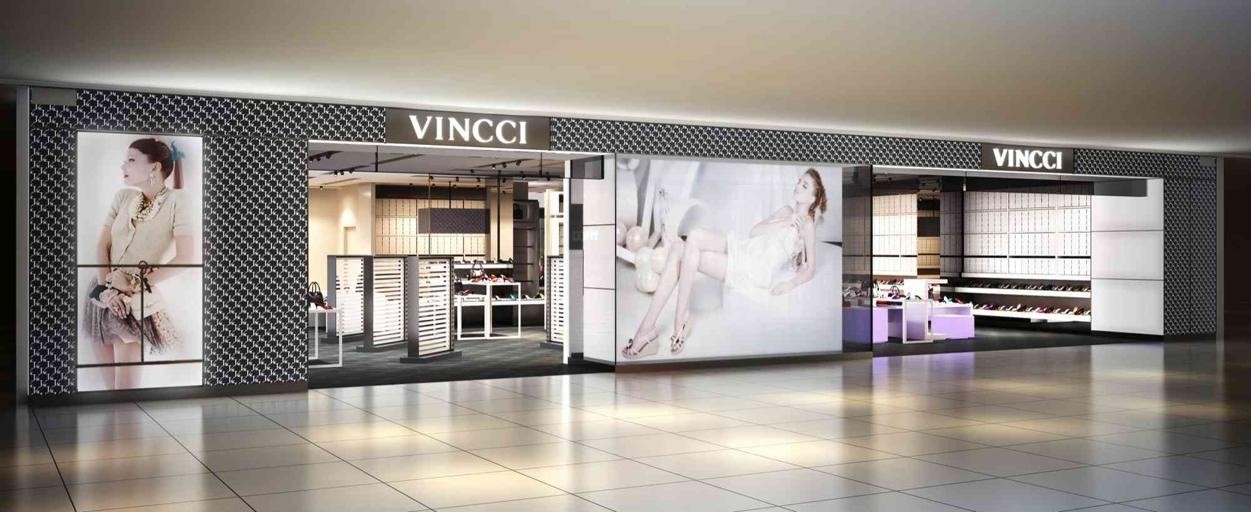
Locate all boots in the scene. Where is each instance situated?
[929,291,933,298]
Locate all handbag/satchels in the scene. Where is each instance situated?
[307,282,324,306]
[129,286,166,321]
[468,261,485,280]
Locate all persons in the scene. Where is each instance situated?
[622,168,830,360]
[84,138,196,390]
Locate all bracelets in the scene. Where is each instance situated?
[128,276,143,294]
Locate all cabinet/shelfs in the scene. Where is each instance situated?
[871,283,1091,323]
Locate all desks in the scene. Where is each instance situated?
[306,308,343,369]
[454,281,521,340]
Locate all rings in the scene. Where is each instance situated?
[107,281,112,284]
[114,306,121,311]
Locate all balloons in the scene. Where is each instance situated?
[623,227,649,252]
[617,222,626,247]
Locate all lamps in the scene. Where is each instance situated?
[330,165,368,176]
[308,151,342,164]
[409,159,561,189]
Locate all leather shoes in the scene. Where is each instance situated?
[323,298,332,309]
[842,277,904,298]
[494,293,544,300]
[968,279,1091,292]
[973,303,1091,316]
[459,257,513,265]
[905,292,922,301]
[938,295,967,304]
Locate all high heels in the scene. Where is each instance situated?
[472,274,512,282]
[671,320,692,353]
[622,335,658,359]
[461,287,475,295]
